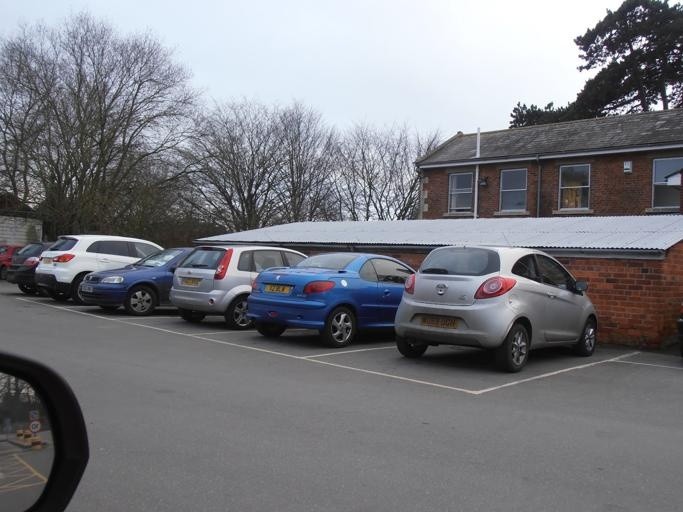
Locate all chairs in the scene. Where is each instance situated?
[261,258,275,270]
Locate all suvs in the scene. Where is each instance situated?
[35,235,175,306]
[169,244,310,330]
[5,242,55,295]
[0,241,25,281]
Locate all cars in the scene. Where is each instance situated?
[393,245,598,374]
[245,252,418,349]
[78,247,192,316]
